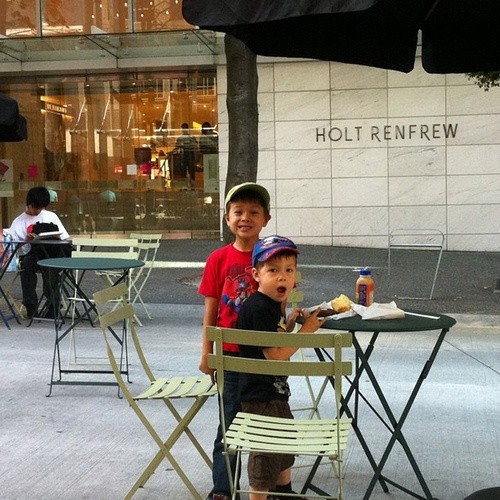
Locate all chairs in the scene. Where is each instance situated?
[11,233,353,499]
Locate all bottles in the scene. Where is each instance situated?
[355,270,374,307]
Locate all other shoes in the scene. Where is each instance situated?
[26,305,39,318]
[265,489,308,500]
[45,306,62,319]
[205,490,240,500]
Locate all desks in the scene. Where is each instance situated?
[35,258,145,400]
[293,309,456,500]
[0,239,72,322]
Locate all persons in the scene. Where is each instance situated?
[232,235,331,500]
[175,121,219,184]
[199,182,273,500]
[9,186,70,319]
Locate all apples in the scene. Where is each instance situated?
[331,294,350,313]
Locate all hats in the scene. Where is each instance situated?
[224,182,271,214]
[252,235,300,268]
